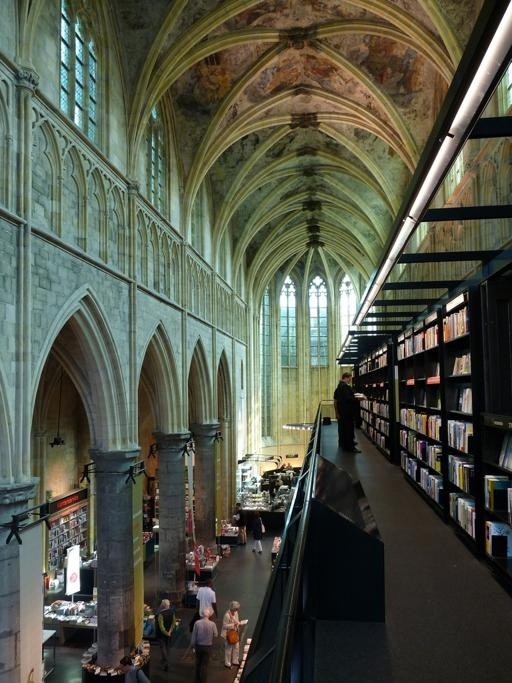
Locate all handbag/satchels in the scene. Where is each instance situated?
[260,517,265,533]
[226,628,240,645]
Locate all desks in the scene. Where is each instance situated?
[58,624,97,647]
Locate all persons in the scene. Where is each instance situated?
[119,578,248,683]
[233,503,265,553]
[280,463,293,473]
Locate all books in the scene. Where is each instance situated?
[43,505,181,676]
[153,480,189,521]
[185,543,231,571]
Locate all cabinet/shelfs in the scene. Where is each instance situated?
[48,506,87,572]
[353,245,511,598]
[43,629,56,680]
